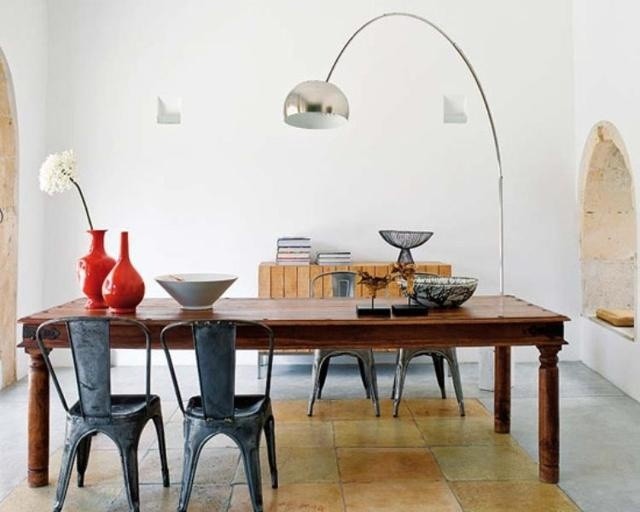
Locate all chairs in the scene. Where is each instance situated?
[307,271,381,417]
[391,273,465,417]
[159,318,278,512]
[36,316,169,512]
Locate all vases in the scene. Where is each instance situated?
[101,232,145,314]
[75,229,117,309]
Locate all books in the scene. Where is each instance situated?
[274,237,352,266]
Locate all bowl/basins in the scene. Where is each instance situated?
[155,273,238,310]
[398,276,480,309]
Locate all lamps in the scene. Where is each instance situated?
[281,11,515,392]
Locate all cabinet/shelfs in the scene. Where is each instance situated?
[257,260,451,380]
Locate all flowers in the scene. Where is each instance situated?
[39,148,94,229]
